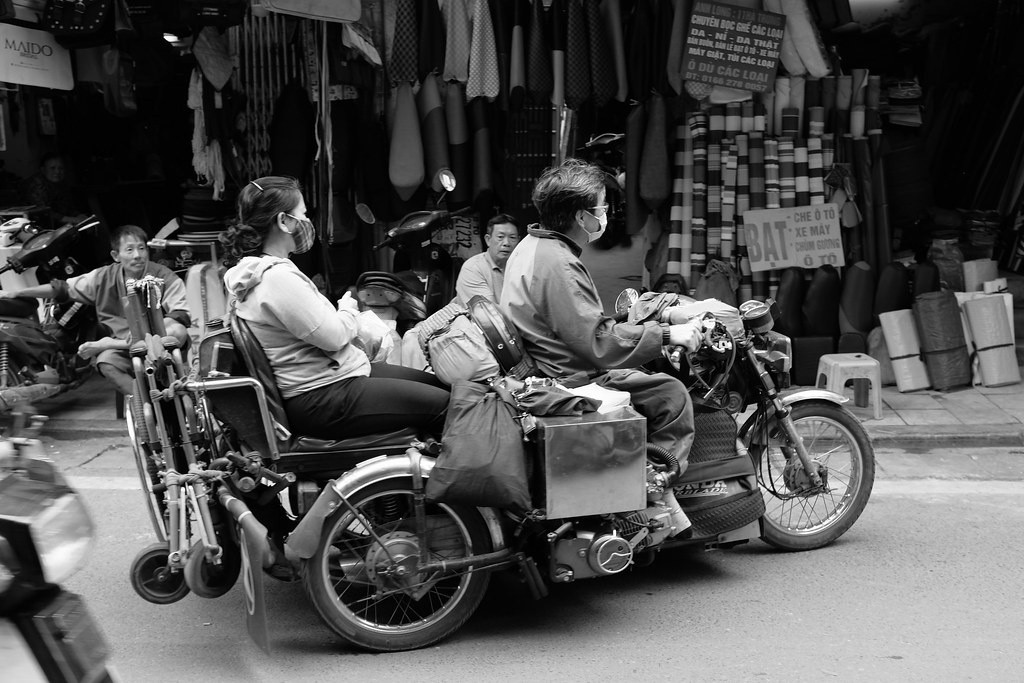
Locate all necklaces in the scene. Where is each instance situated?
[261,252,272,256]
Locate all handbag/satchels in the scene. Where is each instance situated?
[424,378,535,515]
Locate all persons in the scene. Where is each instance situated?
[219,177,451,438]
[0,227,192,431]
[500,159,704,537]
[455,215,517,312]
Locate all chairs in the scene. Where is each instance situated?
[233,310,424,461]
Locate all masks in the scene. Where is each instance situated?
[283,214,316,254]
[579,210,607,245]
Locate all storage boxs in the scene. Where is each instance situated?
[532,405,647,519]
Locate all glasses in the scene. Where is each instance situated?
[588,202,609,214]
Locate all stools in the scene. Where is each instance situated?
[814,353,883,419]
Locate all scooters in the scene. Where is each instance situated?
[0,411,114,683]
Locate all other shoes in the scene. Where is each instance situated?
[672,482,728,498]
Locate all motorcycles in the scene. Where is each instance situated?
[126,273,874,656]
[0,214,109,437]
[354,167,471,339]
